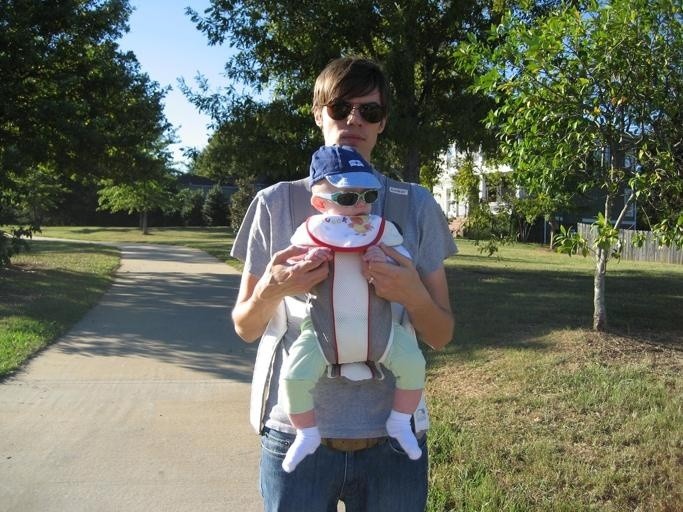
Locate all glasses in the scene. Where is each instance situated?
[321,97,385,123]
[311,190,379,207]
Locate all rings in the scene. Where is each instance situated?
[367,276,374,285]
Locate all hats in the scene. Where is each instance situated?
[309,144,383,190]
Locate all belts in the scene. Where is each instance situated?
[315,435,384,453]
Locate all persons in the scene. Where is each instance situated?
[277,144,427,474]
[228,58,463,512]
